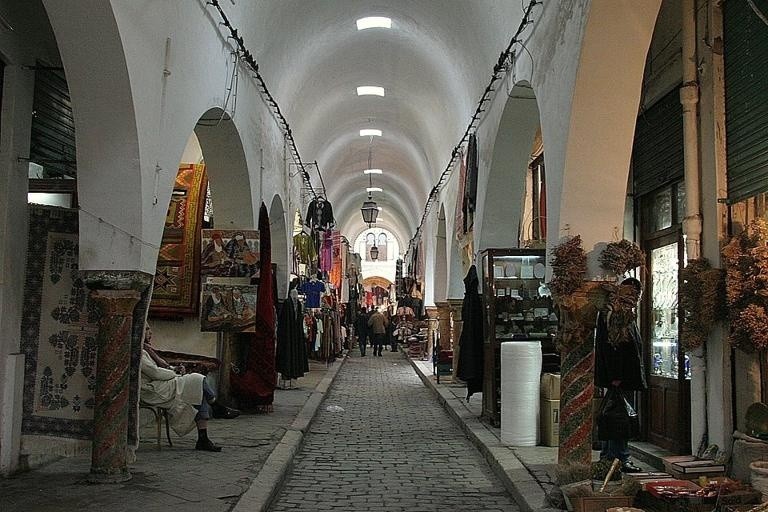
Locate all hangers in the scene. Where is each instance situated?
[472,253,477,265]
[309,273,319,283]
[317,190,325,199]
[293,223,310,238]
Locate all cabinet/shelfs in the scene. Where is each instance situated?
[478,248,560,429]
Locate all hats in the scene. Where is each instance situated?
[360,307,366,313]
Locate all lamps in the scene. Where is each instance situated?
[359,151,378,227]
[369,235,382,262]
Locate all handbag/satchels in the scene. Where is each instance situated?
[595,385,641,442]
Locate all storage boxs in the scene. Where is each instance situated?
[540,371,561,400]
[540,399,560,448]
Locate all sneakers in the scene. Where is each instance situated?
[194,438,222,453]
[213,405,240,419]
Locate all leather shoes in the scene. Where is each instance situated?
[619,459,643,473]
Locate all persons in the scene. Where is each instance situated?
[355,305,399,357]
[139,320,240,452]
[276,289,310,378]
[594,278,648,472]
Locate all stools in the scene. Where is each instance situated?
[140,399,174,447]
